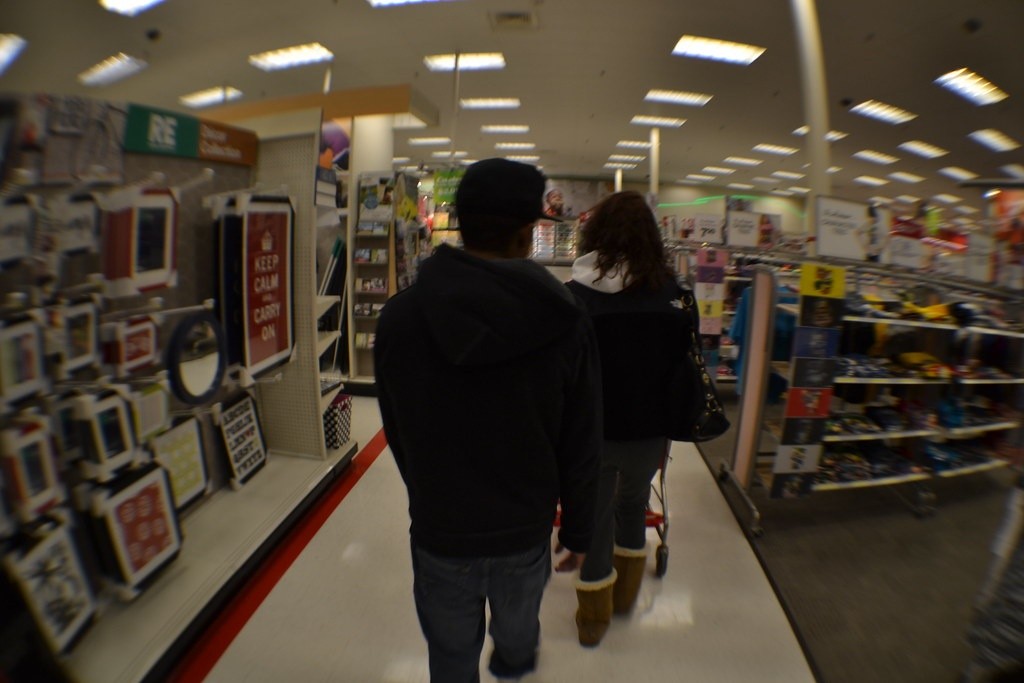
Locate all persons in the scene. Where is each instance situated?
[544,190,565,217]
[373,158,605,683]
[564,190,700,648]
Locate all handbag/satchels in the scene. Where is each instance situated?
[666,272,731,442]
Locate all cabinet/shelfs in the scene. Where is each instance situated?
[0,85,464,683]
[662,235,1024,541]
[528,219,584,267]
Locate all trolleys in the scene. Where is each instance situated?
[554,437,671,578]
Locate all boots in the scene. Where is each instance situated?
[575,565,618,648]
[612,541,647,620]
